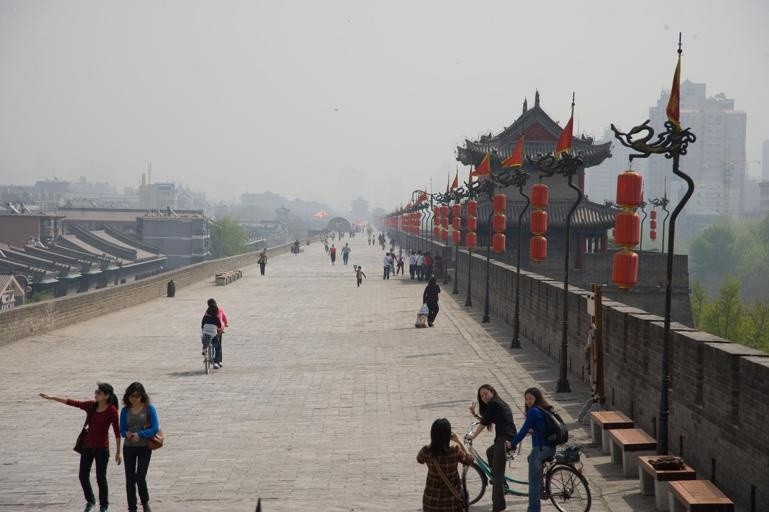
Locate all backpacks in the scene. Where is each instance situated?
[536,404,568,445]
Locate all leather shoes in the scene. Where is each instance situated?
[128,505,137,512]
[84,500,96,512]
[142,501,151,512]
[100,504,108,512]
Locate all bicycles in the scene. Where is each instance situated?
[458,420,592,512]
[200,326,217,376]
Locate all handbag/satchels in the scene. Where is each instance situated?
[420,306,429,314]
[144,401,163,449]
[73,428,89,453]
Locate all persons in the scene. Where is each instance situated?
[38,382,121,511]
[415,417,475,510]
[203,306,220,355]
[423,276,440,329]
[502,387,567,512]
[470,385,518,510]
[257,252,268,277]
[290,229,442,283]
[124,381,160,512]
[354,264,367,286]
[207,298,228,367]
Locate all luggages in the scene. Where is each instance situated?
[415,303,428,328]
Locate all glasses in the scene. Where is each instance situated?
[95,389,104,395]
[128,393,140,398]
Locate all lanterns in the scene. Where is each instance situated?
[612,213,646,247]
[527,180,556,208]
[529,211,549,236]
[529,236,549,262]
[650,219,657,230]
[650,230,658,240]
[378,193,506,254]
[612,165,644,211]
[649,209,657,218]
[613,246,638,286]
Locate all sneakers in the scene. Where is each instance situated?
[214,363,220,369]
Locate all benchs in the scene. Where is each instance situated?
[637,455,697,511]
[665,478,736,512]
[589,410,635,455]
[607,427,659,478]
[215,269,242,286]
